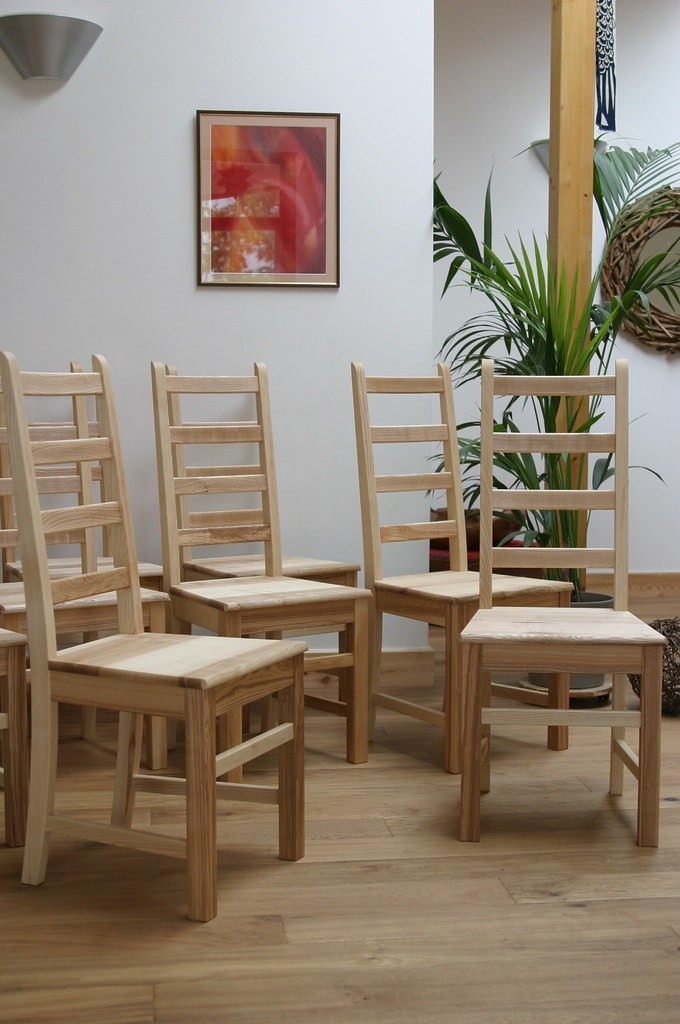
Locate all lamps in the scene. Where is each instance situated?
[0,13,104,84]
[530,138,609,174]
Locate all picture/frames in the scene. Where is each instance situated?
[196,109,340,288]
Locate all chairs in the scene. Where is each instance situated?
[151,362,373,785]
[0,363,172,778]
[455,357,668,849]
[0,460,163,632]
[351,361,574,776]
[0,353,308,923]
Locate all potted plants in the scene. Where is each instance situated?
[433,130,680,690]
